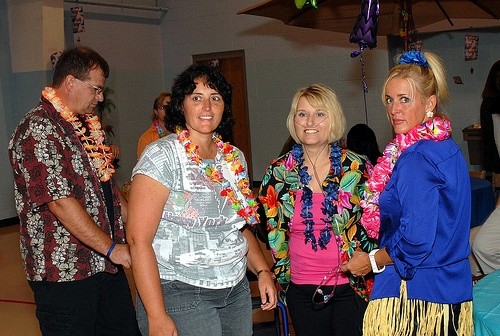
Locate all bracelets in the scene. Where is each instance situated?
[257,269,271,277]
[106,240,117,258]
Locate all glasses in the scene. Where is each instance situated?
[65,74,104,95]
[156,106,167,111]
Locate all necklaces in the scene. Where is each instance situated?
[153,117,171,139]
[359,116,453,237]
[42,87,114,182]
[176,125,259,225]
[293,141,340,250]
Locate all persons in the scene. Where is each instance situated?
[125,64,279,336]
[136,91,175,163]
[256,84,375,336]
[341,50,476,336]
[472,61,500,274]
[8,45,143,336]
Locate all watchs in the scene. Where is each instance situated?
[369,249,385,273]
